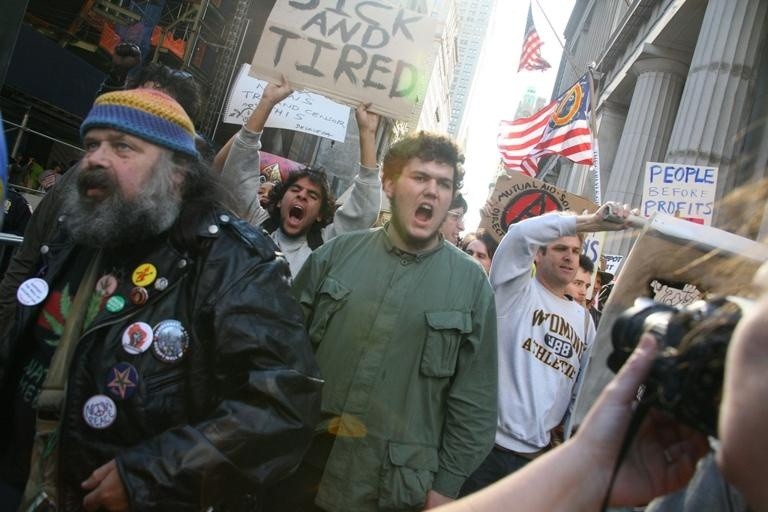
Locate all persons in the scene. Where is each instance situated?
[0,43,767,512]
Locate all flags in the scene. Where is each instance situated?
[516,0,553,70]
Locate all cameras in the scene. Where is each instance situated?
[606,295,753,427]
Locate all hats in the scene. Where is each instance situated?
[79,86,201,161]
